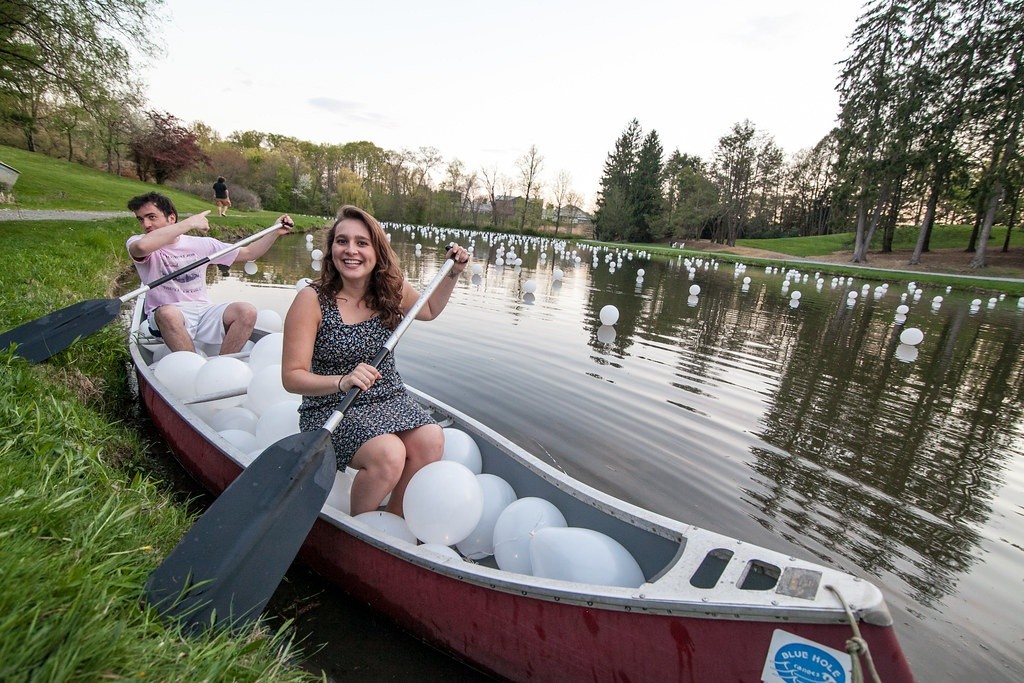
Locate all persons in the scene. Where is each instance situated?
[282,206,470,518]
[213,176,230,217]
[126,191,293,356]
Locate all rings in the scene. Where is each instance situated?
[468,253,471,256]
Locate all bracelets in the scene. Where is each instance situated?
[338,374,347,393]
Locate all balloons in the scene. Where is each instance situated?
[530,528,645,588]
[140,307,394,514]
[456,474,517,559]
[245,236,1024,361]
[403,462,484,547]
[354,511,418,546]
[297,278,313,292]
[900,328,924,345]
[420,542,462,562]
[600,306,619,326]
[493,498,568,575]
[442,429,482,476]
[248,216,1024,313]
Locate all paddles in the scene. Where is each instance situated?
[1,218,296,366]
[136,245,467,640]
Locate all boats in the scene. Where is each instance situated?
[126,279,921,683]
